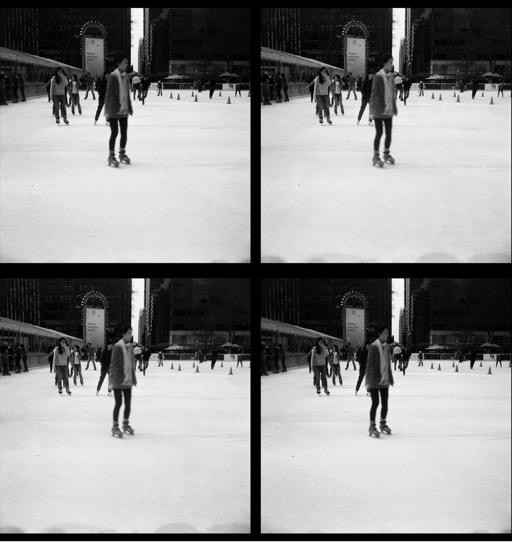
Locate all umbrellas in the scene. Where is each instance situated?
[164,344,196,354]
[217,71,242,80]
[424,343,452,350]
[425,72,460,81]
[480,342,501,348]
[481,71,502,79]
[163,72,196,81]
[219,341,241,354]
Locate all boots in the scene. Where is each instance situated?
[324,386,329,395]
[119,147,131,165]
[122,418,134,433]
[55,116,61,126]
[66,386,71,396]
[317,385,321,395]
[380,418,391,432]
[326,116,332,125]
[108,150,119,168]
[319,115,323,125]
[63,116,70,126]
[372,150,384,168]
[58,385,63,395]
[383,147,395,165]
[111,421,123,435]
[369,421,380,435]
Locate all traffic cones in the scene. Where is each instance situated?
[195,365,200,372]
[191,92,195,97]
[226,97,232,105]
[453,92,456,98]
[487,366,491,375]
[438,93,443,101]
[176,93,181,101]
[455,364,459,372]
[452,361,454,367]
[169,92,174,99]
[177,363,182,371]
[456,95,461,102]
[219,91,222,97]
[193,95,199,102]
[228,367,234,376]
[192,361,196,368]
[430,362,435,368]
[431,92,435,100]
[437,364,442,370]
[220,362,223,366]
[489,98,494,105]
[170,362,173,369]
[479,361,482,367]
[480,92,484,98]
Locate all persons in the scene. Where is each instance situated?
[0,335,163,396]
[364,324,395,438]
[468,349,477,370]
[104,54,135,166]
[497,78,505,99]
[470,78,479,100]
[209,351,218,370]
[233,78,242,98]
[261,64,425,128]
[495,349,502,368]
[369,53,397,166]
[0,65,163,125]
[236,351,243,368]
[208,78,216,99]
[261,335,425,396]
[107,323,135,439]
